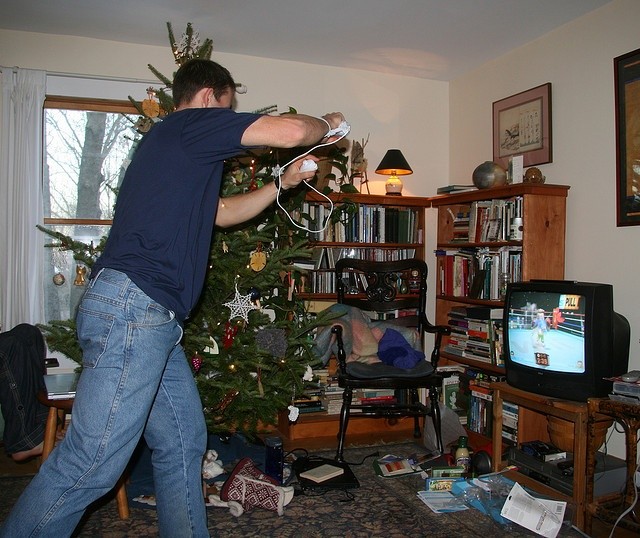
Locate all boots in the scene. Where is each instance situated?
[219,457,295,518]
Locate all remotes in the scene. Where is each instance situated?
[557,460,573,469]
[503,279,630,401]
[622,370,640,383]
[563,466,574,476]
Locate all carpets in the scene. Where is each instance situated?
[2,442,592,537]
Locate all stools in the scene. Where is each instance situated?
[40,392,130,521]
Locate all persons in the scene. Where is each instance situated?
[1,58,346,538]
[0,322,62,465]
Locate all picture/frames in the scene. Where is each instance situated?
[613,47,640,227]
[492,83,552,171]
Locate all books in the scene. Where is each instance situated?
[433,361,519,450]
[284,245,417,295]
[436,184,478,197]
[265,199,422,241]
[361,308,419,323]
[451,195,522,243]
[437,305,504,367]
[289,310,321,357]
[433,246,521,301]
[285,364,408,417]
[417,467,470,514]
[300,463,345,484]
[43,371,78,401]
[372,457,427,479]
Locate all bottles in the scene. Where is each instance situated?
[454,436,471,471]
[263,436,283,486]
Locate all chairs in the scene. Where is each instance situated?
[332,258,451,457]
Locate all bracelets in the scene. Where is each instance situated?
[276,176,288,194]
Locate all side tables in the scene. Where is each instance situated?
[585,397,640,538]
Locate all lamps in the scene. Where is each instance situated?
[375,150,412,196]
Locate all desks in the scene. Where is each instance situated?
[489,383,613,533]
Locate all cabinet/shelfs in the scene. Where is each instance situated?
[431,183,568,460]
[277,192,430,456]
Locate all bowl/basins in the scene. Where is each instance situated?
[42,373,75,394]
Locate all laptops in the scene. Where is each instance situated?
[296,460,359,491]
